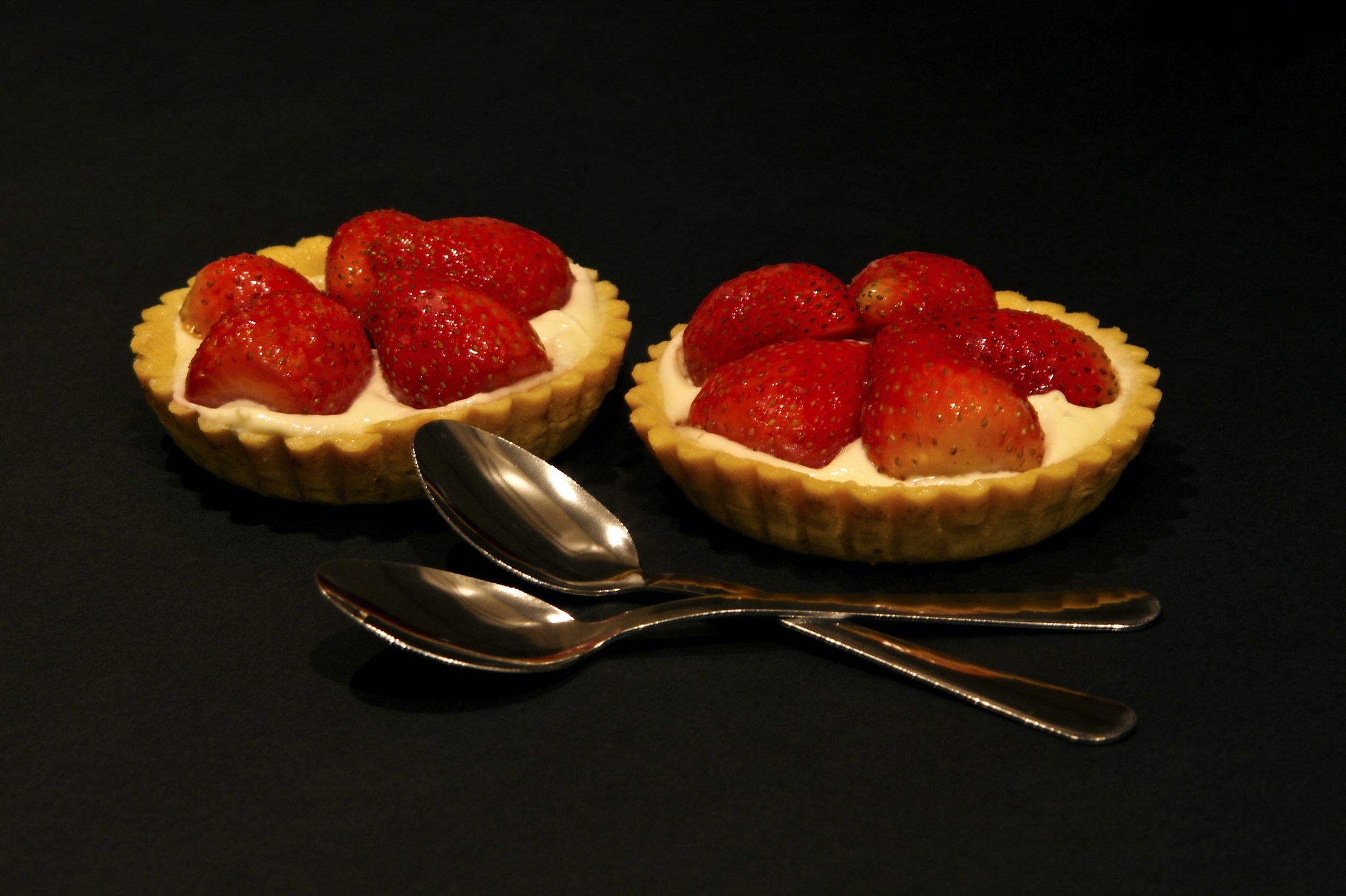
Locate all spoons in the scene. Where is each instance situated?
[312,557,1159,674]
[413,419,1135,741]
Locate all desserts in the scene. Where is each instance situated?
[625,251,1162,563]
[131,213,632,504]
